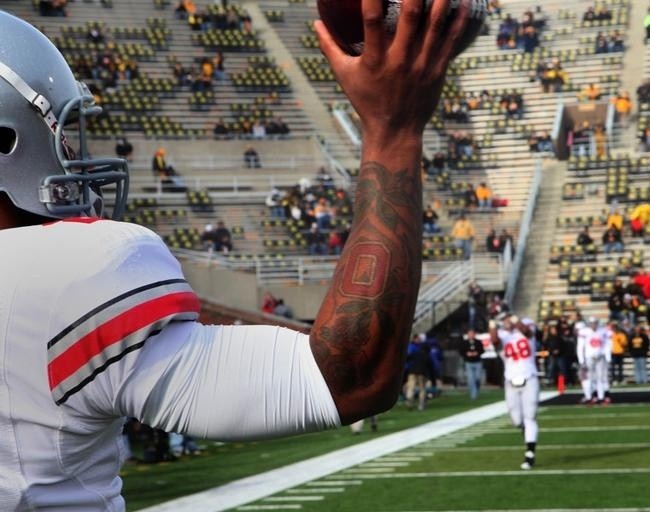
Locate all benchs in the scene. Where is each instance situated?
[413,1,648,361]
[1,1,362,286]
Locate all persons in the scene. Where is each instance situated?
[0,2,470,510]
[487,314,539,468]
[0,1,649,458]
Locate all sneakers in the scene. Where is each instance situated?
[520,450,535,470]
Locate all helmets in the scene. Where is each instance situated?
[0,12,129,221]
[490,302,509,320]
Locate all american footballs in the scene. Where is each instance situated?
[317,0,488,60]
